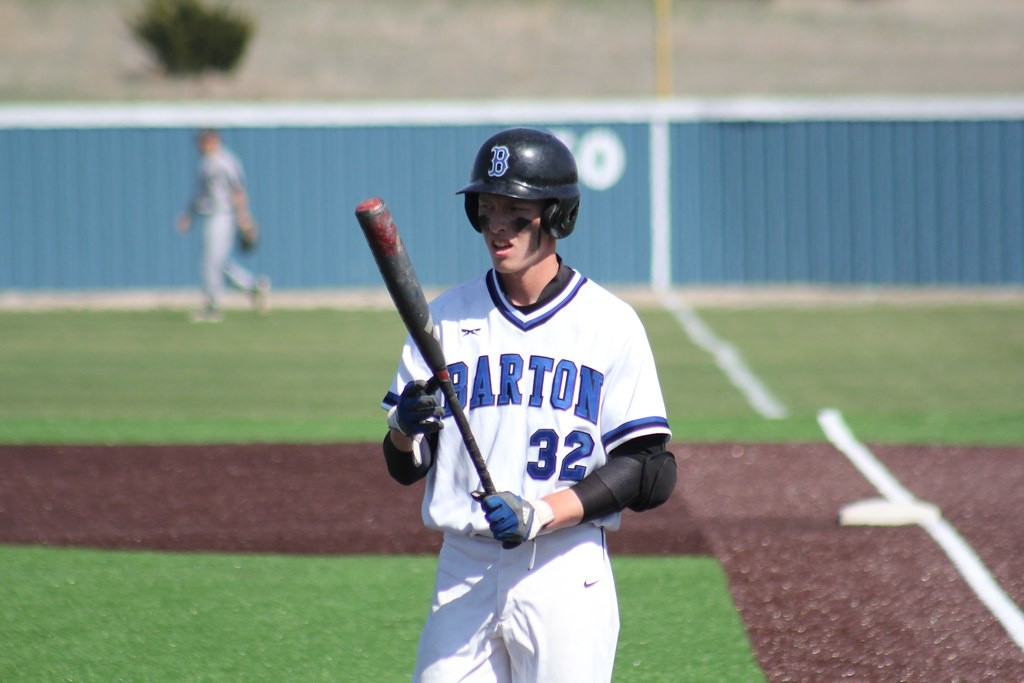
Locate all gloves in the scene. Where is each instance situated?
[469,490,553,549]
[387,377,445,433]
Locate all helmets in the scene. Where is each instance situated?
[456,128,579,239]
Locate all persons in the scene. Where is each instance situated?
[178,129,272,322]
[380,128,678,683]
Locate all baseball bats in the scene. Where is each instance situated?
[356,198,523,551]
